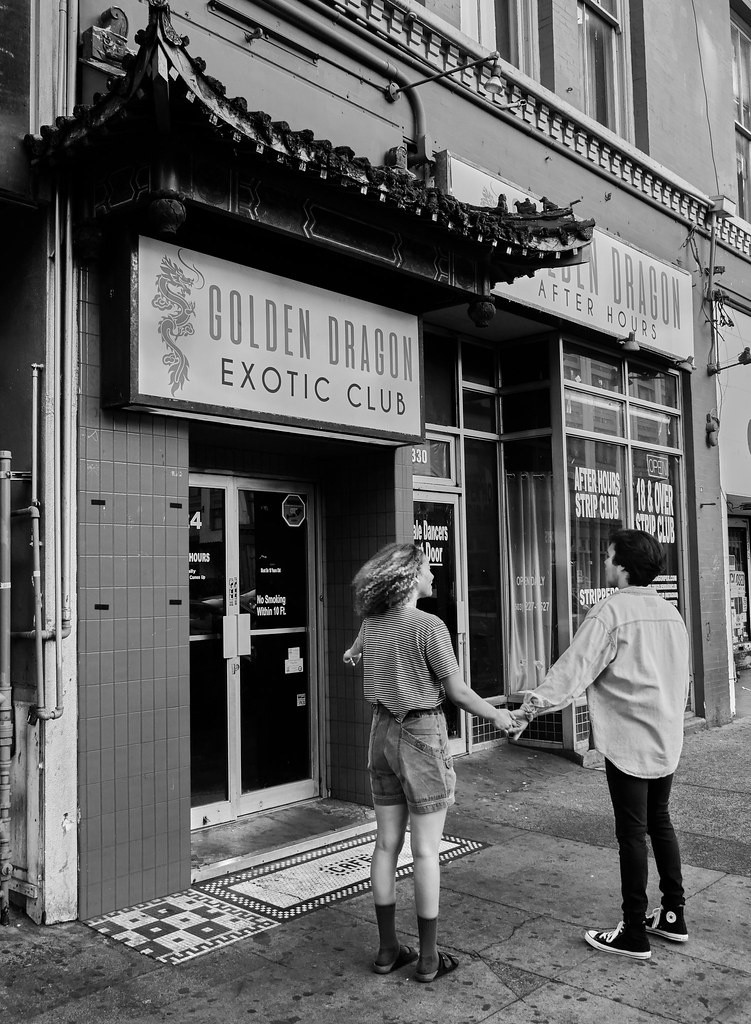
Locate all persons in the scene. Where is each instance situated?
[342,543,519,984]
[508,529,690,959]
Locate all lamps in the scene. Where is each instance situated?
[673,356,697,374]
[385,50,503,104]
[616,331,641,354]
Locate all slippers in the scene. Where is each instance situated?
[373,945,419,974]
[415,950,459,983]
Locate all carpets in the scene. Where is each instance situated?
[82,818,494,966]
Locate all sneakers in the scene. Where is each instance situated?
[584,920,652,960]
[645,904,689,943]
